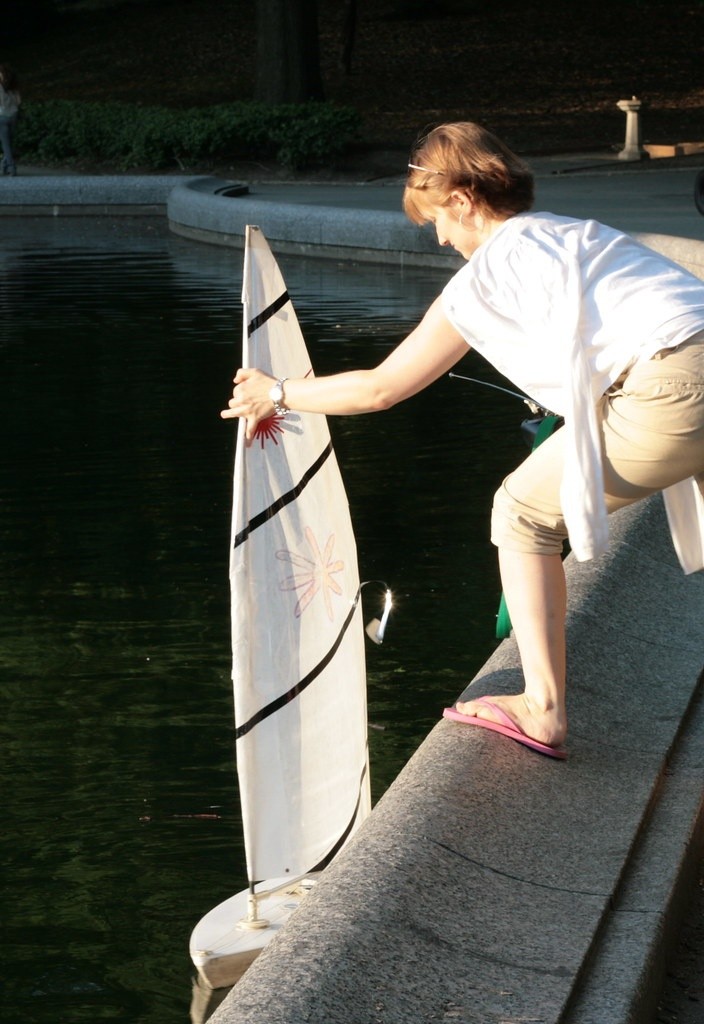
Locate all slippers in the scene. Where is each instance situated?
[442,695,571,758]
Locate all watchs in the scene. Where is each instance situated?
[270,378,288,415]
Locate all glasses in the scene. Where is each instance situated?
[406,156,445,176]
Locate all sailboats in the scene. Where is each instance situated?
[185,224,384,993]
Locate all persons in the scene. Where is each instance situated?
[220,120,703,761]
